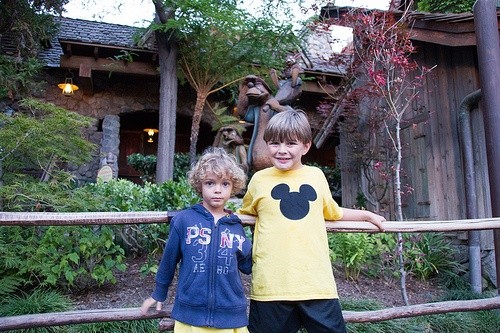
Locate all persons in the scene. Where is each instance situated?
[234,106,389,333]
[140,147,256,333]
